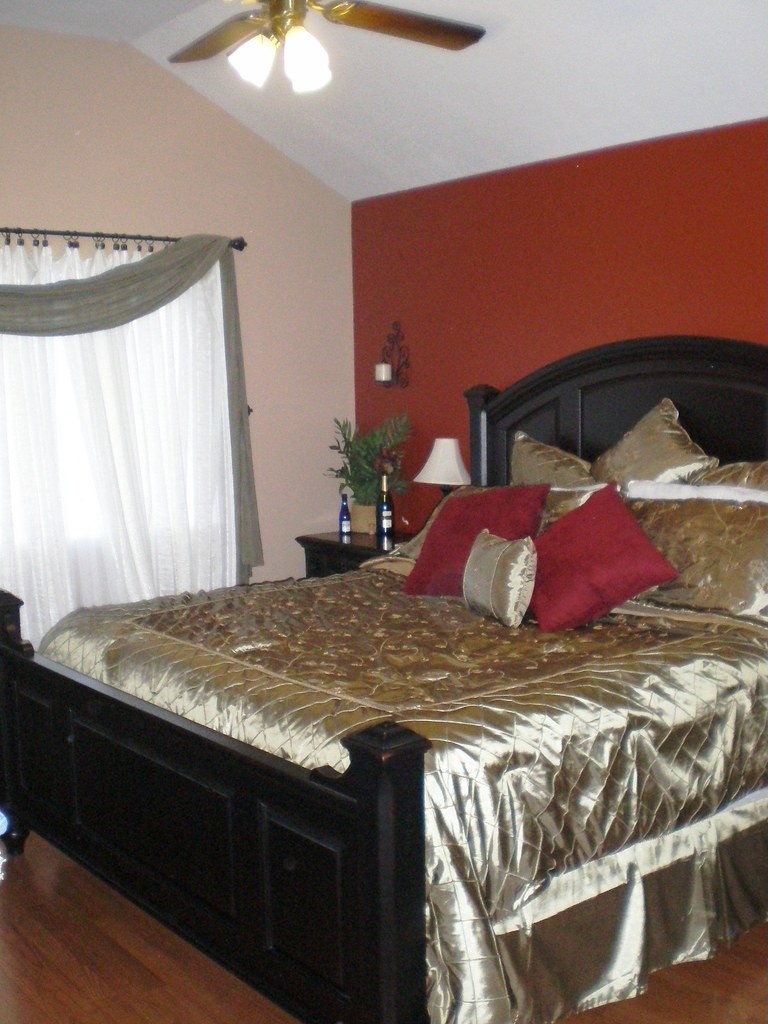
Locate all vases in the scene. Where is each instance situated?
[351,503,378,535]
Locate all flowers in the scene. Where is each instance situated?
[325,410,413,507]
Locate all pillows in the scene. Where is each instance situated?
[460,526,538,627]
[626,480,767,502]
[361,489,589,577]
[404,484,549,596]
[621,492,768,623]
[588,398,722,486]
[512,432,595,486]
[688,460,768,490]
[522,480,680,632]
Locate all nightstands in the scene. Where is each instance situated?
[295,529,415,583]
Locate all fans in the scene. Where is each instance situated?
[168,1,487,64]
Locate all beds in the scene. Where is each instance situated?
[0,335,768,1024]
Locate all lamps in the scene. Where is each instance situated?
[371,322,409,390]
[413,439,474,496]
[231,25,332,92]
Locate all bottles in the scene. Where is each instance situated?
[339,494,352,534]
[376,472,394,552]
